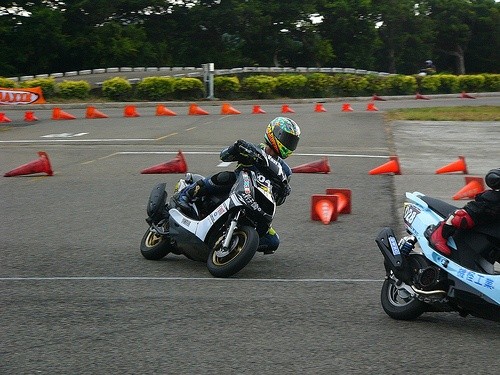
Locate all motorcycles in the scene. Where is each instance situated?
[138,139,295,275]
[373,190,500,322]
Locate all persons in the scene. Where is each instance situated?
[425,60,436,74]
[176,117,301,255]
[429,168,500,256]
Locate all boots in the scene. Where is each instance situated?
[429,209,473,255]
[170,177,210,212]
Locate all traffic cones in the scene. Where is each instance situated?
[310,193,338,225]
[325,188,352,216]
[452,177,484,201]
[435,155,467,174]
[3,151,52,177]
[368,157,401,178]
[280,104,376,112]
[141,149,187,174]
[124,105,139,117]
[86,106,108,118]
[24,111,39,121]
[156,104,176,116]
[188,103,209,115]
[52,107,75,120]
[291,155,330,175]
[253,105,265,113]
[0,112,12,123]
[221,103,241,114]
[372,91,474,101]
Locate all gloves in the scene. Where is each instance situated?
[276,178,291,197]
[228,139,248,156]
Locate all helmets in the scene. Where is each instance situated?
[264,117,300,160]
